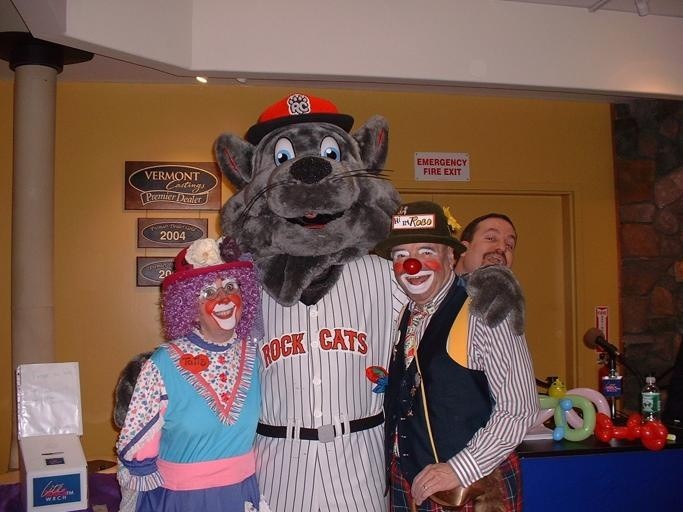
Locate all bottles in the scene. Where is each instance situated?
[641,374,662,423]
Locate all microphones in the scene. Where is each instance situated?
[583,327,621,357]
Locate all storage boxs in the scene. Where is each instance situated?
[16,434,89,512]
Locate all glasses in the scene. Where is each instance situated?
[199,281,241,301]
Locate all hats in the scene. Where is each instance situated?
[157,243,256,294]
[242,90,356,146]
[372,200,467,261]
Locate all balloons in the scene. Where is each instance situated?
[526,378,669,451]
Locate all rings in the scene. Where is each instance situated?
[423,484,428,490]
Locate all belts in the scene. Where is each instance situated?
[255,409,385,442]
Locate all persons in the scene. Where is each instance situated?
[372,202,540,512]
[112,241,272,512]
[452,214,518,276]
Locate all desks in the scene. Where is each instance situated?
[514,431,683,512]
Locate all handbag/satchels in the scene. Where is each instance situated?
[426,482,476,507]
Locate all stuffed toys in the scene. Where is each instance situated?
[112,94,526,512]
